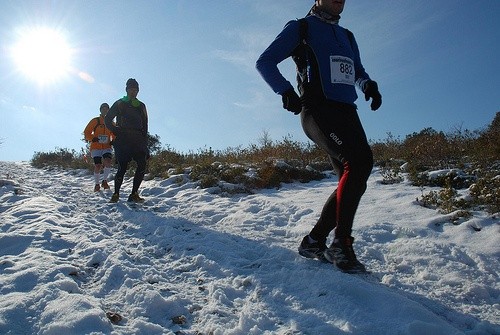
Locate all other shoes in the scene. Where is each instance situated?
[110,193,119,205]
[128,194,144,203]
[95,184,100,193]
[102,180,110,189]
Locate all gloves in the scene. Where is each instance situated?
[112,127,120,135]
[282,89,302,115]
[93,138,99,142]
[145,149,150,160]
[109,141,114,145]
[365,81,382,110]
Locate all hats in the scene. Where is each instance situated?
[126,79,139,90]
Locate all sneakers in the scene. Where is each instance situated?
[298,235,329,262]
[324,241,365,273]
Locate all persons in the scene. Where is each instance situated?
[84,103,116,193]
[105,78,150,204]
[255,0,382,274]
